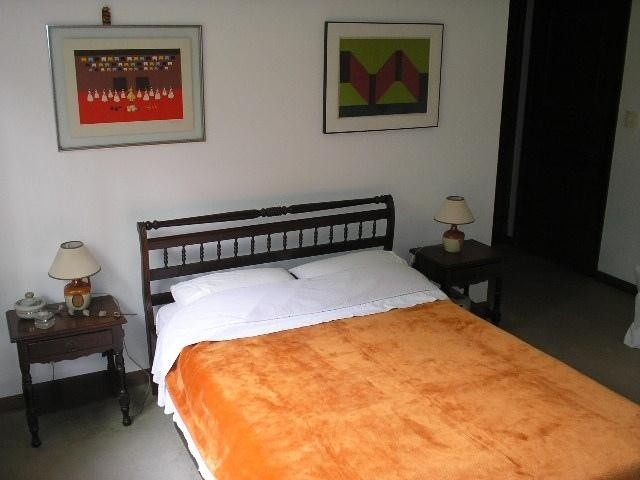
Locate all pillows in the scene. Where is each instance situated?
[169,249,412,303]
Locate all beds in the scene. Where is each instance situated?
[136,193,639,479]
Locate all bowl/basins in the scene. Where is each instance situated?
[14,292,46,319]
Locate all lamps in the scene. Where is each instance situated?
[49,240,101,312]
[434,195,476,253]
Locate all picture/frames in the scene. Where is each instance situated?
[45,24,207,151]
[323,20,444,135]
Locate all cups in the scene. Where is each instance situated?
[34,311,55,329]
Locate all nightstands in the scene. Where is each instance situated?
[409,240,503,327]
[5,295,133,447]
